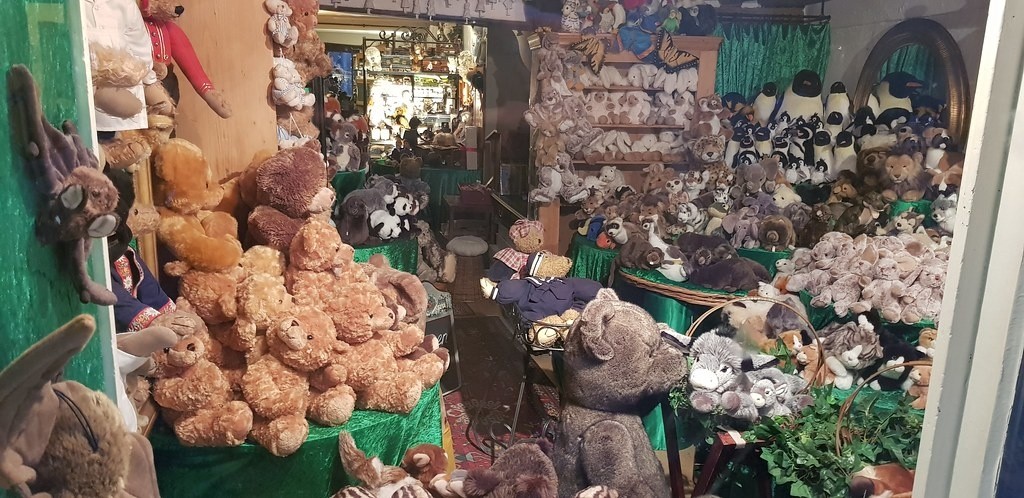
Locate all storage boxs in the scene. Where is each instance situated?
[464,126,478,170]
[458,183,492,204]
[355,43,450,72]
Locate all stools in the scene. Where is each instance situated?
[445,256,489,316]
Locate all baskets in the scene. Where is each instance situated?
[835,360,932,459]
[671,297,826,440]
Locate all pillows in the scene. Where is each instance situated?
[446,235,488,256]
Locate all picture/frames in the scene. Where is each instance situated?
[499,162,529,195]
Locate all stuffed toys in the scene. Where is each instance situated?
[322,91,457,284]
[0,314,162,498]
[524,0,722,204]
[5,0,331,330]
[331,218,687,497]
[574,69,964,324]
[137,216,451,456]
[685,296,937,498]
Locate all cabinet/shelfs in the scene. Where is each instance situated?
[354,69,459,144]
[525,31,724,252]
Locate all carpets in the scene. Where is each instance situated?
[441,316,564,473]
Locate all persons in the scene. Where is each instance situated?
[403,117,428,145]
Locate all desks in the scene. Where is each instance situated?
[439,192,494,248]
[331,169,367,203]
[154,381,444,498]
[799,290,936,345]
[354,237,418,277]
[369,159,480,229]
[738,247,792,279]
[563,233,620,285]
[618,265,749,456]
